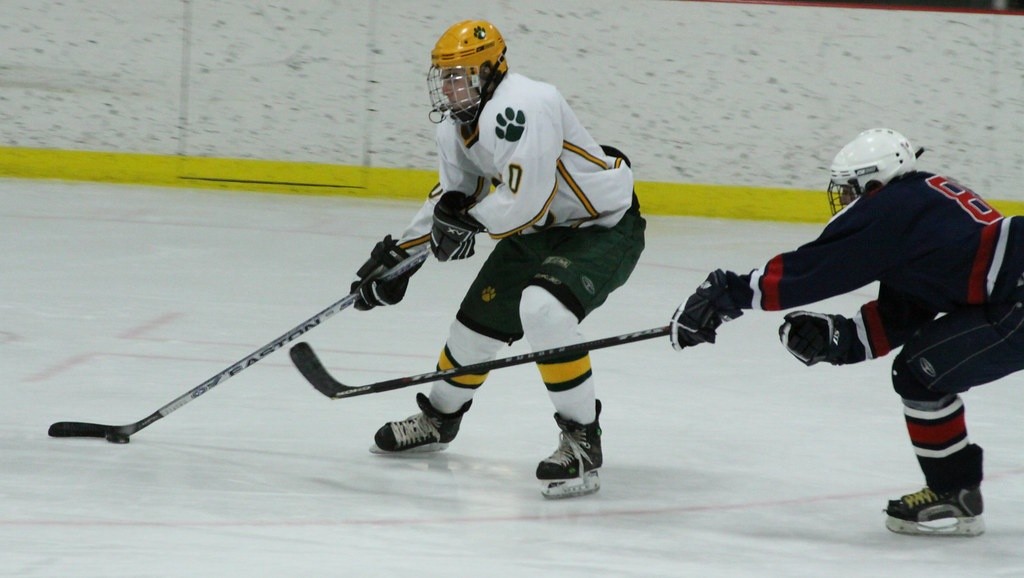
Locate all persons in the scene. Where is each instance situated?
[349,18,647,498]
[668,129,1024,535]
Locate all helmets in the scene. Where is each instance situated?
[430,19,509,78]
[830,128,917,198]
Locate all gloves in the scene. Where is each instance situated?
[429,191,486,261]
[670,268,760,351]
[778,308,866,365]
[348,234,424,311]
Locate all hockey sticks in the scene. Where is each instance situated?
[50,246,438,441]
[287,324,671,399]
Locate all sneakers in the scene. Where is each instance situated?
[884,443,986,534]
[369,391,472,454]
[535,398,602,497]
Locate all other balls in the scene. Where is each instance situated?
[105,434,131,444]
